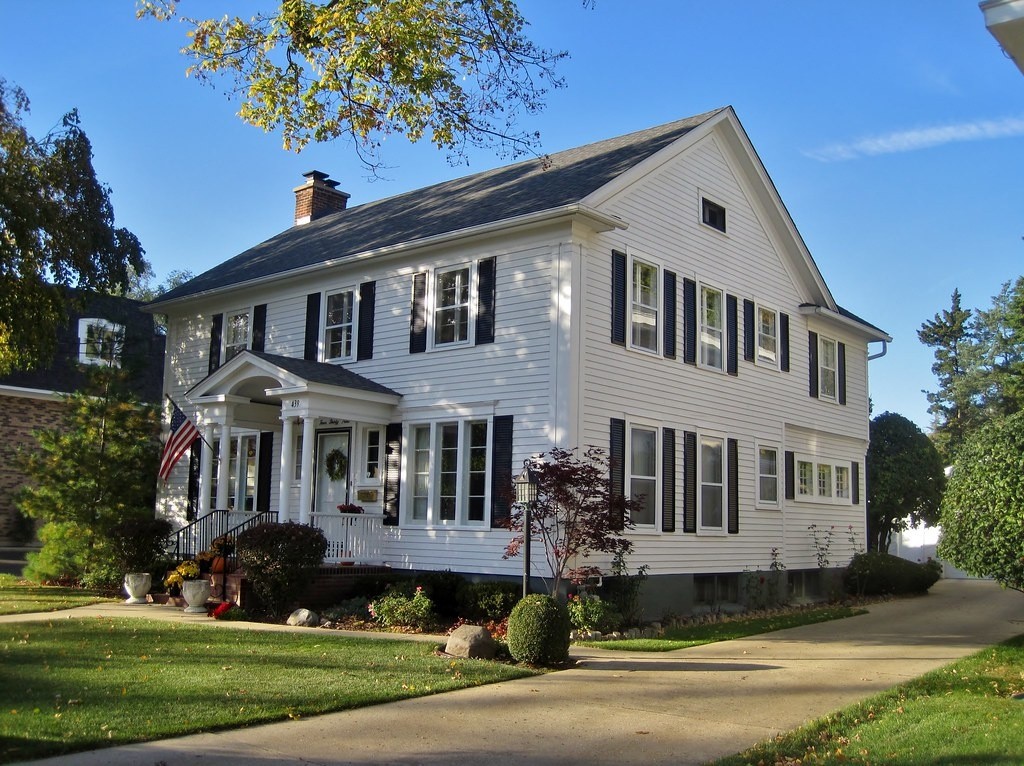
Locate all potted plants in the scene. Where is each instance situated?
[337,503,365,526]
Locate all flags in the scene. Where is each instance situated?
[158,405,199,486]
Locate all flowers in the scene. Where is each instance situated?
[846,523,880,608]
[365,585,436,631]
[163,570,184,591]
[195,550,216,562]
[210,533,233,551]
[808,521,837,601]
[176,560,200,580]
[742,547,787,611]
[566,592,622,635]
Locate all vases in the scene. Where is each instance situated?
[218,545,233,556]
[170,586,181,597]
[199,559,211,574]
[181,576,195,582]
[181,579,210,613]
[124,572,153,604]
